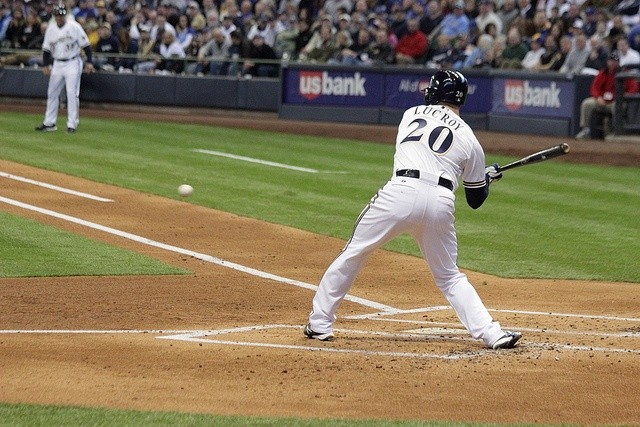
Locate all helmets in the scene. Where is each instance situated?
[53,3,67,15]
[421,69,468,105]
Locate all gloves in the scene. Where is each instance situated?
[486,163,503,181]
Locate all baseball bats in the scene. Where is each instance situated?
[499,142,569,171]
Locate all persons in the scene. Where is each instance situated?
[575,55,627,141]
[35,7,93,131]
[1,0,70,70]
[304,68,522,351]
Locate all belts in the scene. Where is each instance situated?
[396,170,452,190]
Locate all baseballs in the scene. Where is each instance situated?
[178,184,193,197]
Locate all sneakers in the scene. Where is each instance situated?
[574,127,591,140]
[303,323,334,341]
[493,331,522,350]
[34,124,58,132]
